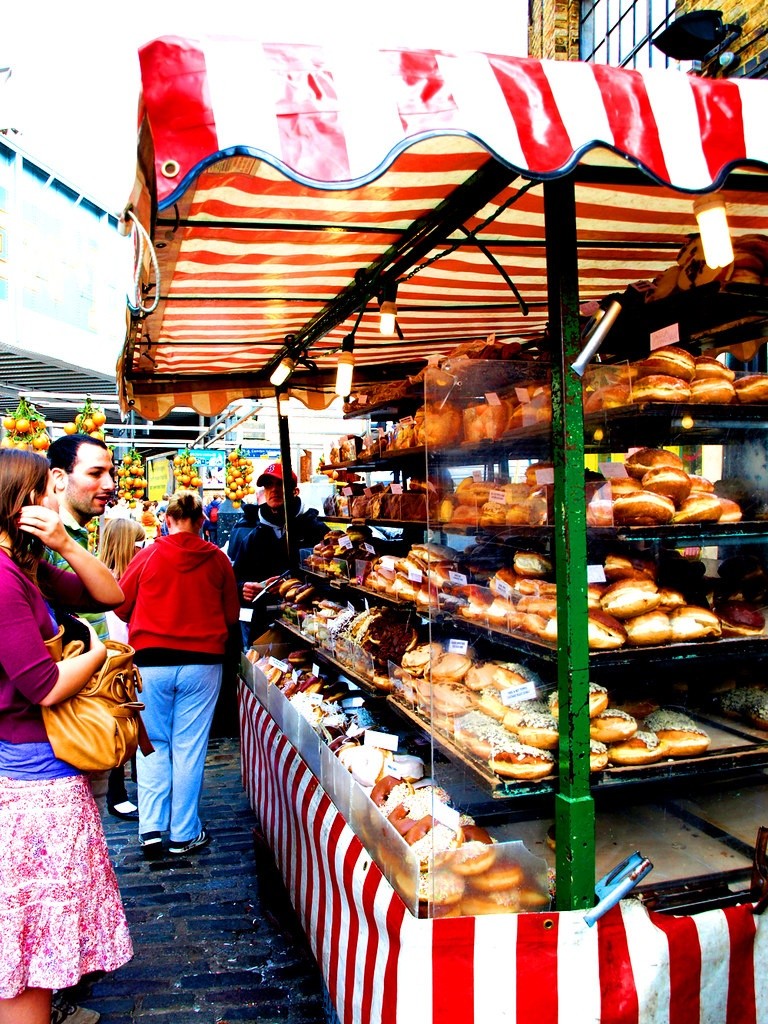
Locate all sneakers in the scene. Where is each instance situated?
[50,991,100,1024]
[138,831,162,846]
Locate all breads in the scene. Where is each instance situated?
[246,230,767,919]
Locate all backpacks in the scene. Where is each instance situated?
[210,502,219,523]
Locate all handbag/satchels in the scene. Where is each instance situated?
[40,622,155,773]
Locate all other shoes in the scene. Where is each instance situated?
[169,826,209,854]
[107,800,139,821]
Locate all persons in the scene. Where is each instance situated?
[0,448,135,1024]
[43,434,332,1024]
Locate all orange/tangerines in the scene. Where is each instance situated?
[224,451,255,509]
[85,522,95,551]
[173,455,203,492]
[117,456,147,510]
[0,412,113,457]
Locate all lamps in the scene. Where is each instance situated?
[650,10,743,63]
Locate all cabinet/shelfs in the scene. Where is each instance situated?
[275,279,768,800]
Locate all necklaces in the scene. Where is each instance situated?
[0,545,13,553]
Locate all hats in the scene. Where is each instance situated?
[257,463,298,489]
